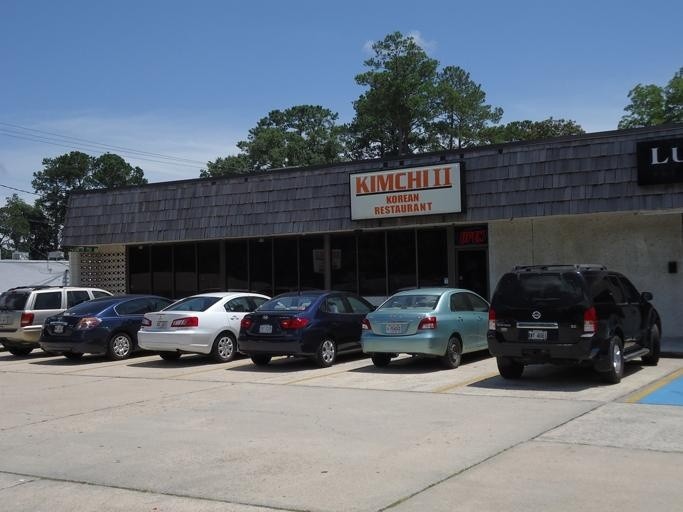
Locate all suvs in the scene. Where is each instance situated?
[486,264,661,384]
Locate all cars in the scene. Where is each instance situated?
[237,290,378,368]
[37,294,176,361]
[136,292,289,363]
[0,285,114,356]
[359,288,491,369]
